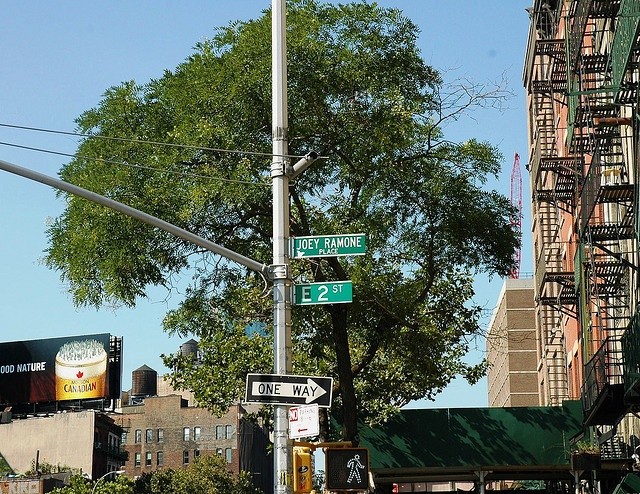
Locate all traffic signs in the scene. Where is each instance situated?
[292,233,365,258]
[244,373,334,409]
[294,281,352,305]
[288,404,319,439]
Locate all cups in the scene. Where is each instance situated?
[54,351,107,399]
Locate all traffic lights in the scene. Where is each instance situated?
[326,447,371,490]
